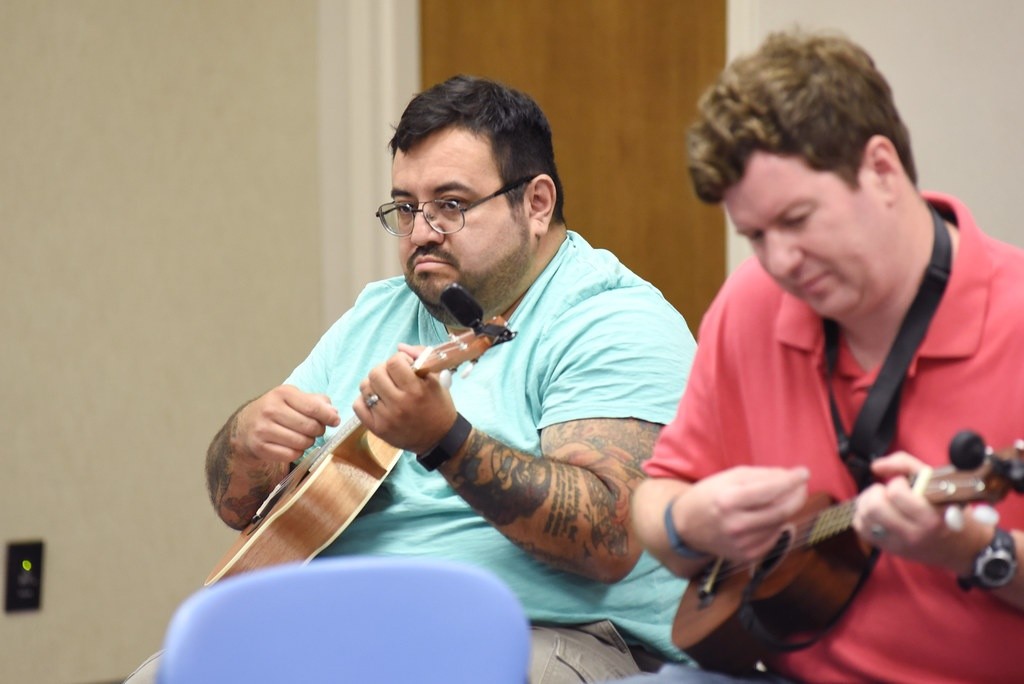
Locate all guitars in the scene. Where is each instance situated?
[668,431,1023,675]
[206,310,511,587]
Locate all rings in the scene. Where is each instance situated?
[365,394,379,408]
[870,524,887,539]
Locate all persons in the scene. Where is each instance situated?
[611,29,1024,684]
[123,74,699,684]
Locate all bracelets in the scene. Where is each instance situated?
[664,496,711,560]
[416,412,472,472]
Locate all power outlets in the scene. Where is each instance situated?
[5,540,46,613]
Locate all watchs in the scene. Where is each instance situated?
[958,528,1018,592]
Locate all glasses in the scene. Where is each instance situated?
[380,174,538,237]
